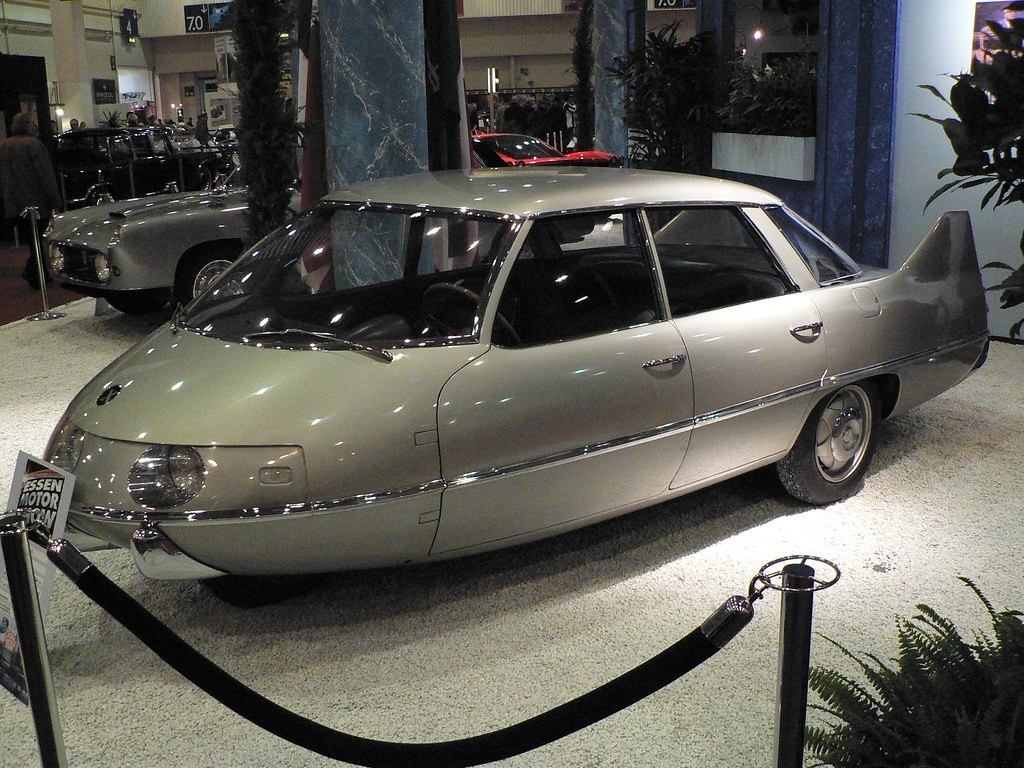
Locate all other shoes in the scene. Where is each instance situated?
[21,266,40,290]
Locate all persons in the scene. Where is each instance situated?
[464,89,581,153]
[0,113,62,290]
[51,111,211,145]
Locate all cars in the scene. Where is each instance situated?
[45,167,991,610]
[214,121,306,179]
[43,161,302,317]
[471,133,625,169]
[53,126,223,206]
